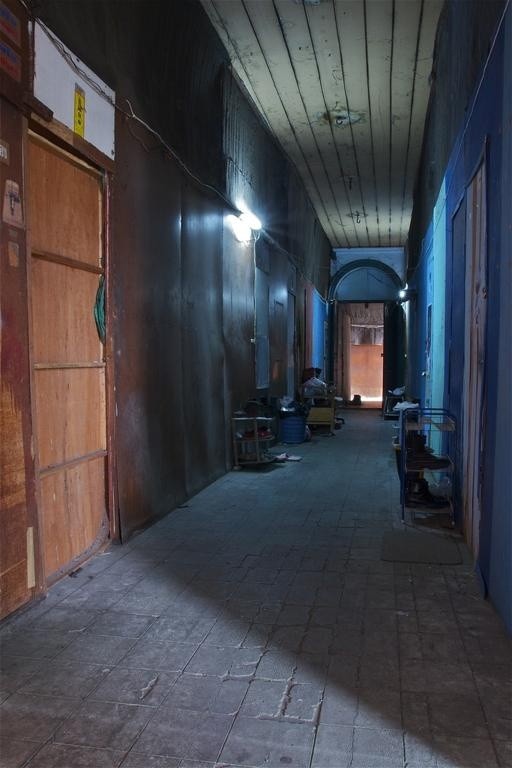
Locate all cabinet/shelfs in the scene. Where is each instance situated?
[392,404,422,446]
[384,390,406,420]
[232,418,276,466]
[403,413,458,519]
[302,383,337,433]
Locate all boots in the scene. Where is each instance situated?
[405,478,450,509]
[406,432,450,470]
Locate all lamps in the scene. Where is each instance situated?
[226,207,261,244]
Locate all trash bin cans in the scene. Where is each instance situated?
[279,398,309,443]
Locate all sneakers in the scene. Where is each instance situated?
[244,429,273,440]
[393,401,419,411]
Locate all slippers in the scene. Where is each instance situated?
[276,453,302,461]
